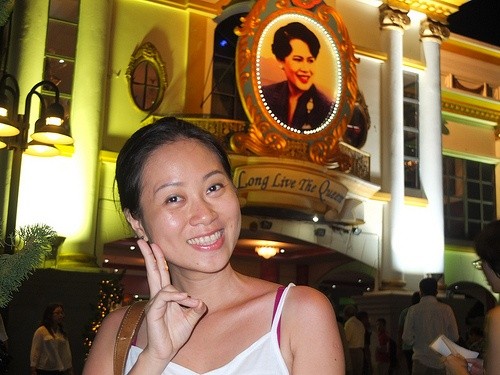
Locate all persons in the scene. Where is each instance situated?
[342,304,365,375]
[261,21,335,130]
[375,317,399,375]
[80,117,347,375]
[0,315,17,375]
[397,292,422,375]
[29,303,74,375]
[438,220,500,375]
[336,311,382,375]
[401,276,460,375]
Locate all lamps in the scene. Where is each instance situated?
[313,212,319,222]
[352,227,362,235]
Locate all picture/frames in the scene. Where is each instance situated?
[227,0,361,173]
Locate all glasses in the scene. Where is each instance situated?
[472,256,489,270]
